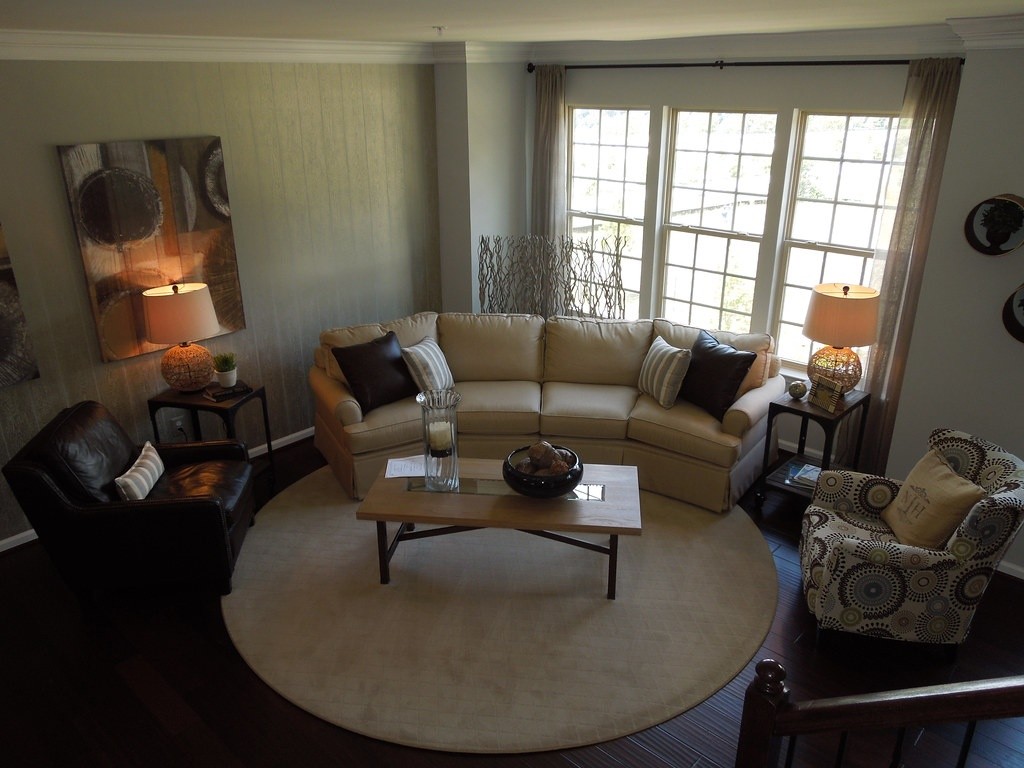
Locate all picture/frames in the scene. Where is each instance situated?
[808,373,843,414]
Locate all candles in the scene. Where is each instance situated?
[425,422,456,458]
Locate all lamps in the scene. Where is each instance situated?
[802,283,880,396]
[143,282,220,392]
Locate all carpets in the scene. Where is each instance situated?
[223,465,777,752]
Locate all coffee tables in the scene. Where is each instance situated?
[357,455,641,599]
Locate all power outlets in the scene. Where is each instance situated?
[170,415,186,437]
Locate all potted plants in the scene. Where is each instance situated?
[213,352,237,388]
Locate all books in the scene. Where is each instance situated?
[765,454,823,499]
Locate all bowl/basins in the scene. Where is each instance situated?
[503,444,584,498]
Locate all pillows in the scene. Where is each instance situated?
[115,439,165,501]
[637,329,758,426]
[881,445,990,550]
[330,331,455,415]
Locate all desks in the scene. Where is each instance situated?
[148,381,273,479]
[757,378,871,507]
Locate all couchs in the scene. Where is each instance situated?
[798,427,1024,649]
[306,311,784,514]
[1,400,264,595]
